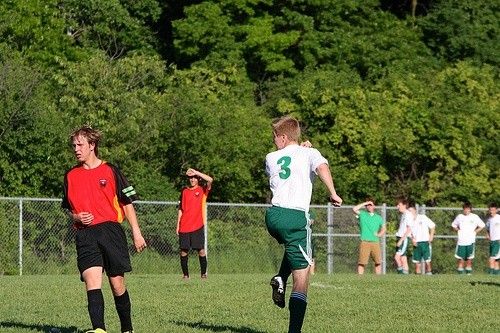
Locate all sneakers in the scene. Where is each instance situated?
[270,275,286,308]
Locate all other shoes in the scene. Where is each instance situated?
[201,274,206,279]
[182,274,189,279]
[84,328,108,333]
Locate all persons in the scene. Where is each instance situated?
[451,201,486,275]
[485,203,500,275]
[394,199,413,274]
[352,198,386,274]
[265,116,342,333]
[406,203,436,275]
[176,168,213,279]
[61,124,147,333]
